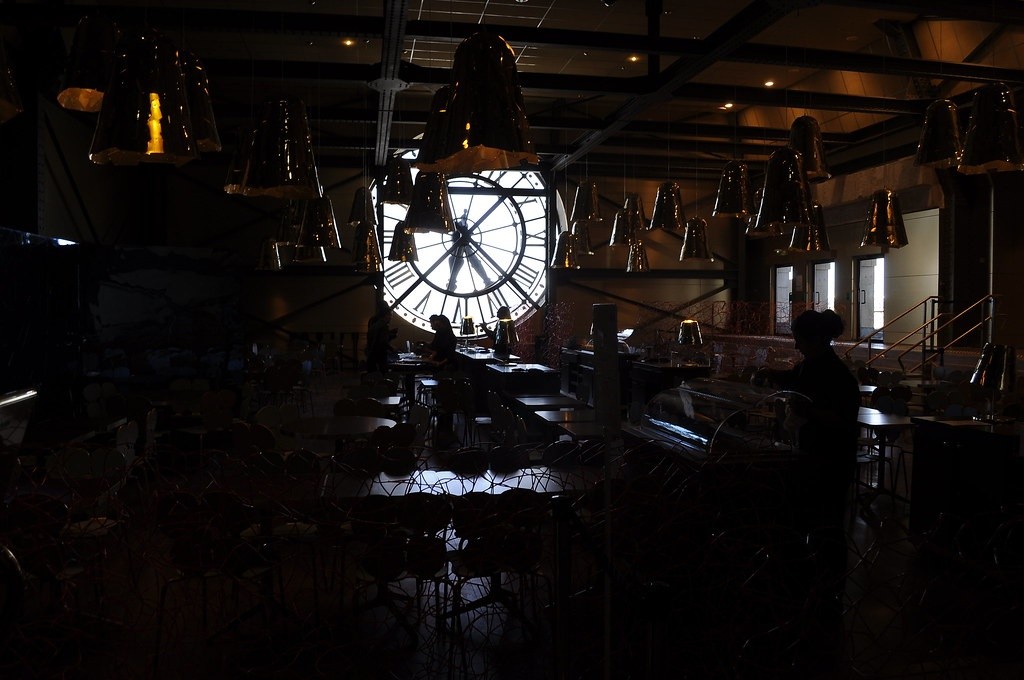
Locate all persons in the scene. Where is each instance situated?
[480,307,516,355]
[419,315,438,353]
[783,308,861,640]
[365,308,401,373]
[367,299,398,343]
[429,315,459,366]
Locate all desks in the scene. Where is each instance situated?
[1,328,1023,680]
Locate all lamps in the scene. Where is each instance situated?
[6,14,1022,279]
